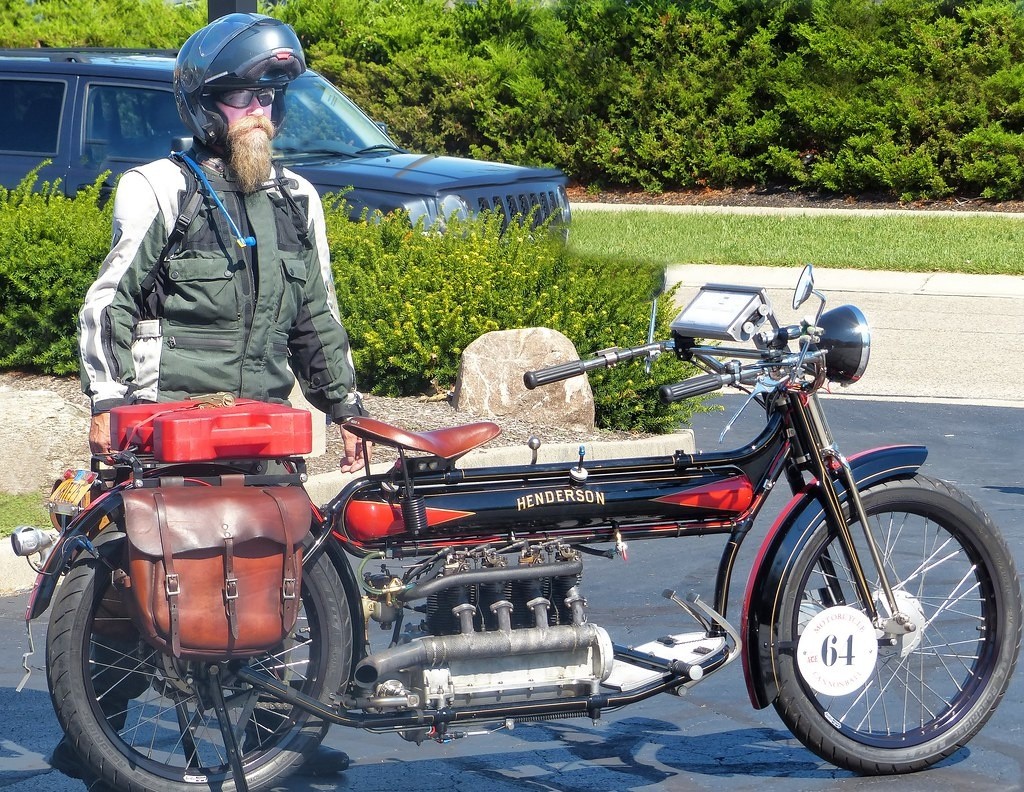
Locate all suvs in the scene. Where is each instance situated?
[0,45,574,255]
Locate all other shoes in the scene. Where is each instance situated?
[243,707,350,774]
[47,695,140,792]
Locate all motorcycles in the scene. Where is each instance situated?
[10,265,1024,792]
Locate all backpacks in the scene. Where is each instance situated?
[120,487,312,660]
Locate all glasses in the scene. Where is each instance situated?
[215,86,276,110]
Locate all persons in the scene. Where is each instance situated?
[49,12,373,792]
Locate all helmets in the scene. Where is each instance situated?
[173,21,307,148]
